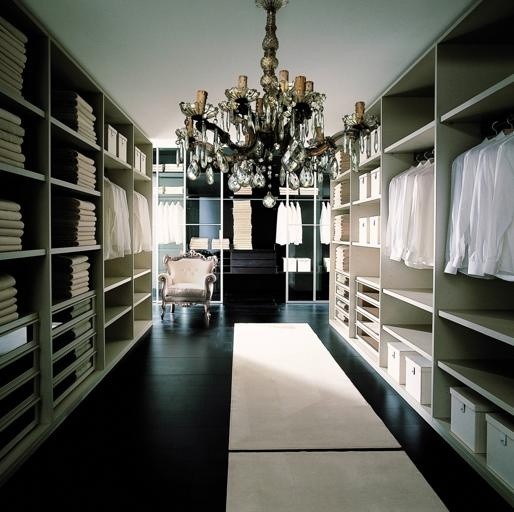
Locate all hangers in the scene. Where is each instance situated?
[482,117,513,136]
[277,197,331,207]
[410,148,435,166]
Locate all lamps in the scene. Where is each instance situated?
[173,0,378,208]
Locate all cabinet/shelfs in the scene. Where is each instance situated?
[348,97,383,367]
[381,40,436,431]
[316,155,332,301]
[105,95,134,349]
[51,29,108,408]
[133,122,156,333]
[1,2,49,472]
[432,0,513,507]
[278,170,315,301]
[184,150,278,319]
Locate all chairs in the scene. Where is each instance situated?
[158,246,219,326]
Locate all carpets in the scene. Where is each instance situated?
[225,447,453,511]
[227,324,402,450]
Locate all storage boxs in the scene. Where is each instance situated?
[403,350,433,407]
[282,256,330,273]
[386,338,420,388]
[358,124,382,248]
[486,413,513,494]
[445,386,491,457]
[106,121,148,172]
